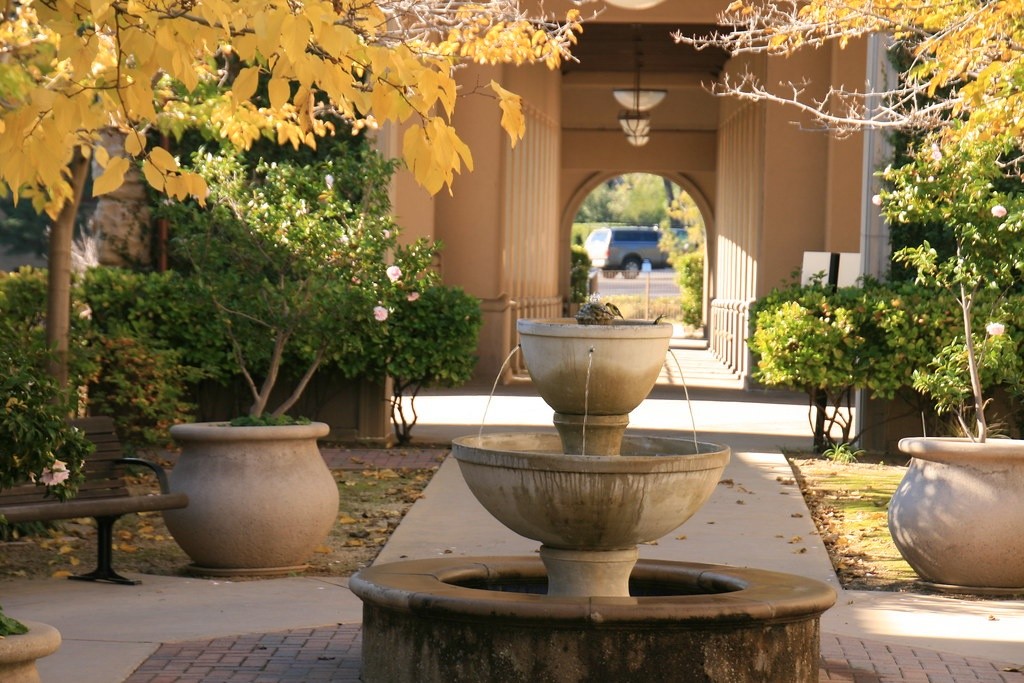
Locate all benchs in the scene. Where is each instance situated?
[0,416,189,586]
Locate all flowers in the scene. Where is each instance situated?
[0,318,97,628]
[140,132,447,421]
[869,140,1024,444]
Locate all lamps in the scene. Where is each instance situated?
[618,108,651,146]
[613,23,667,112]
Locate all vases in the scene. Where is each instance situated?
[888,437,1024,597]
[0,621,62,683]
[158,419,343,577]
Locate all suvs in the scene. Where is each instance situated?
[583,223,669,280]
